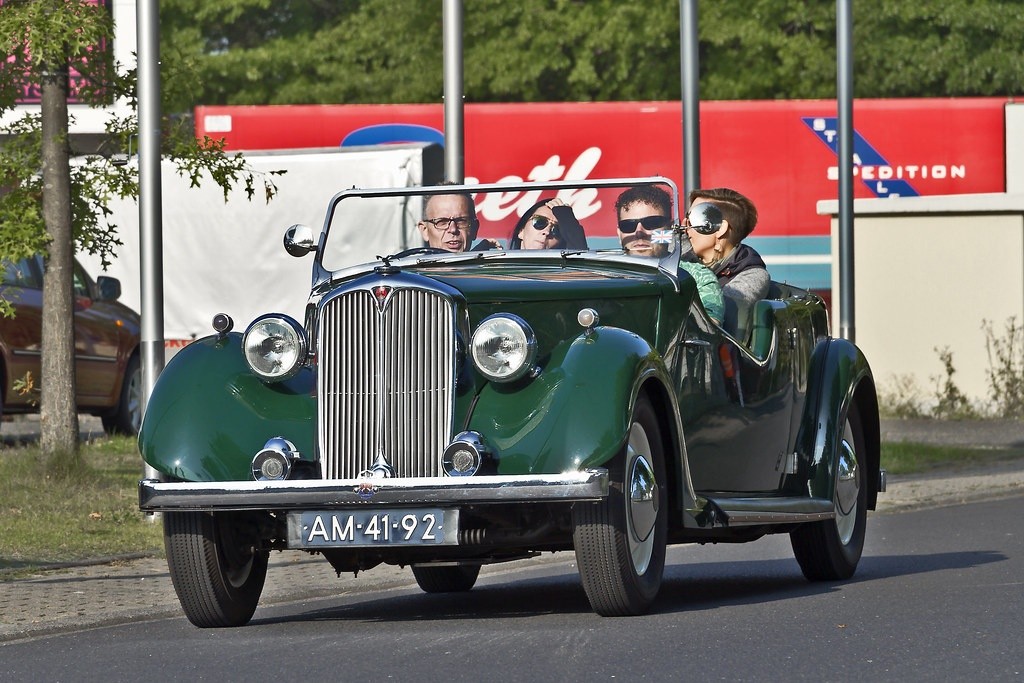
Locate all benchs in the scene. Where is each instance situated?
[750,279,815,361]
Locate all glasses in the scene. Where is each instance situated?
[617,215,670,234]
[424,213,478,229]
[528,214,562,240]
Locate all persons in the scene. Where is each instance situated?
[418,181,480,252]
[614,185,724,328]
[470,197,589,252]
[671,188,771,344]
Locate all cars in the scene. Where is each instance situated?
[142,176,887,628]
[0,254,142,438]
